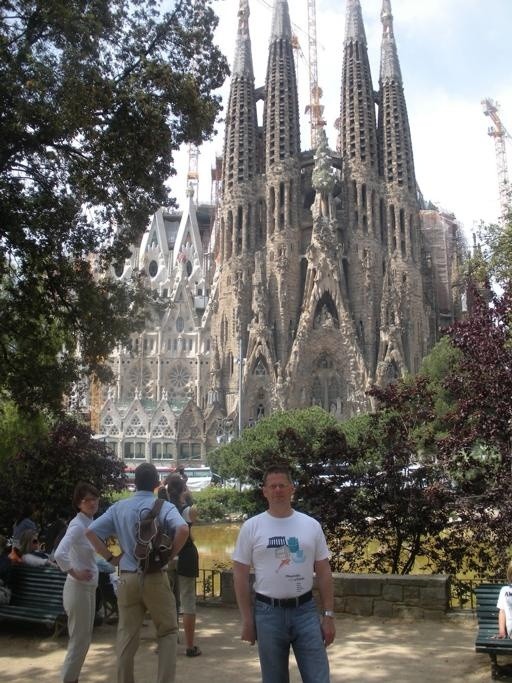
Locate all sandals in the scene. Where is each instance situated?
[187,646,201,658]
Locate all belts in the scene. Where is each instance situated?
[256,590,313,608]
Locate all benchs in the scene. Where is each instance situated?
[0,562,68,639]
[475,583,512,680]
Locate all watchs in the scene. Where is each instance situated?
[322,608,335,618]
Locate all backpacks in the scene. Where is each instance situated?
[177,536,200,579]
[132,498,173,570]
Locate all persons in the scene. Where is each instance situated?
[162,464,202,656]
[85,463,189,682]
[491,561,511,638]
[1,500,123,626]
[54,480,101,682]
[231,463,335,682]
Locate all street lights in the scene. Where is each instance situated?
[234,317,246,434]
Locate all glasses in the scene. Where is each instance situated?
[31,539,38,545]
[263,483,289,490]
